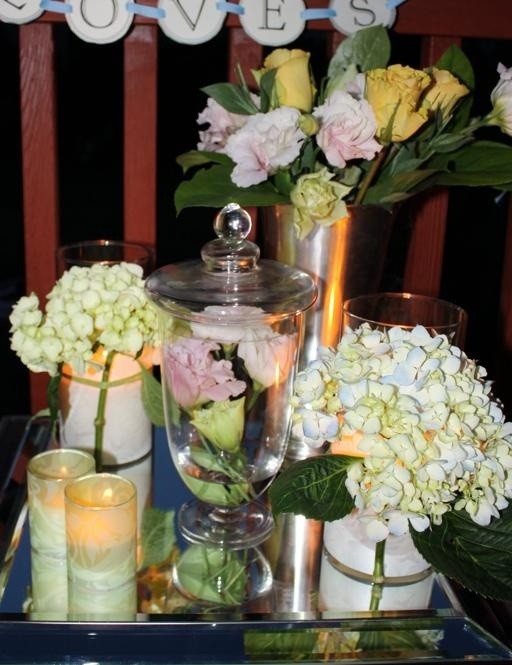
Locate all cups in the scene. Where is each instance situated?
[340,289,469,353]
[51,235,153,284]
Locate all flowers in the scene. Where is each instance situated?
[176,23,510,241]
[295,322,511,600]
[11,261,172,379]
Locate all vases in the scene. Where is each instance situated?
[55,349,153,517]
[276,198,364,370]
[318,502,439,616]
